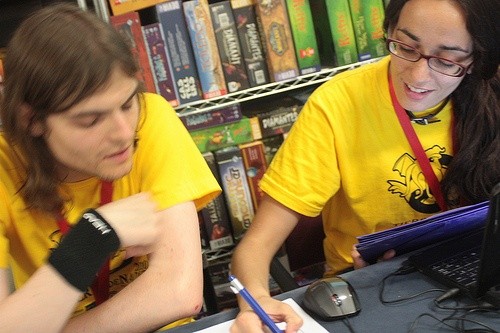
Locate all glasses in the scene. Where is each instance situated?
[386,22,476,78]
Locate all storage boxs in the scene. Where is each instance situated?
[106,0,391,250]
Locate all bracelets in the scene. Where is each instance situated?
[45,208,121,292]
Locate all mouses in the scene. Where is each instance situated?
[301,276,361,322]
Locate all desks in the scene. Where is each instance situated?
[154,256,500,333]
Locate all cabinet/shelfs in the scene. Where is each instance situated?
[94,1,386,270]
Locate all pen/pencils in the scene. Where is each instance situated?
[227,274,283,333]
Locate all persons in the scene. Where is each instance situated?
[0,0,224,333]
[229,0,500,333]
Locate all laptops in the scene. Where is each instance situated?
[408,191,500,300]
